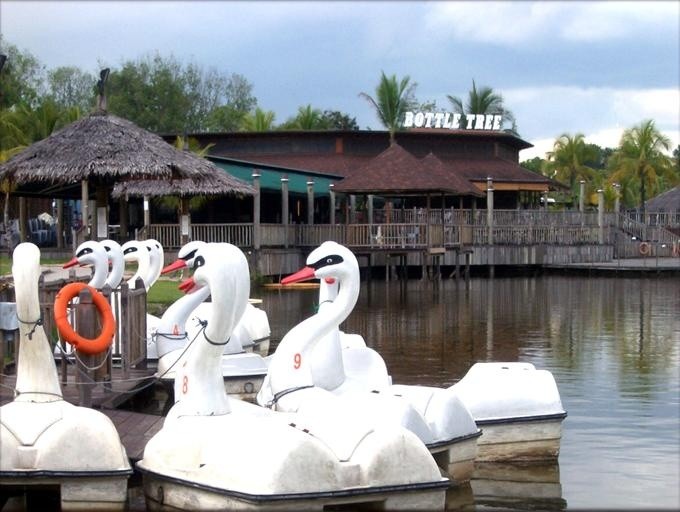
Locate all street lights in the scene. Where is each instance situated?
[597,189,606,244]
[329,183,337,225]
[615,185,621,211]
[486,177,494,244]
[579,180,585,210]
[251,172,263,248]
[280,177,290,249]
[544,191,548,209]
[306,181,316,226]
[612,182,616,188]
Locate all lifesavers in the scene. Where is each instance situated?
[639,242,650,254]
[53,281,115,353]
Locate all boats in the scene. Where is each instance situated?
[136,257,451,512]
[251,241,484,486]
[52,236,276,395]
[0,241,135,503]
[315,275,568,466]
